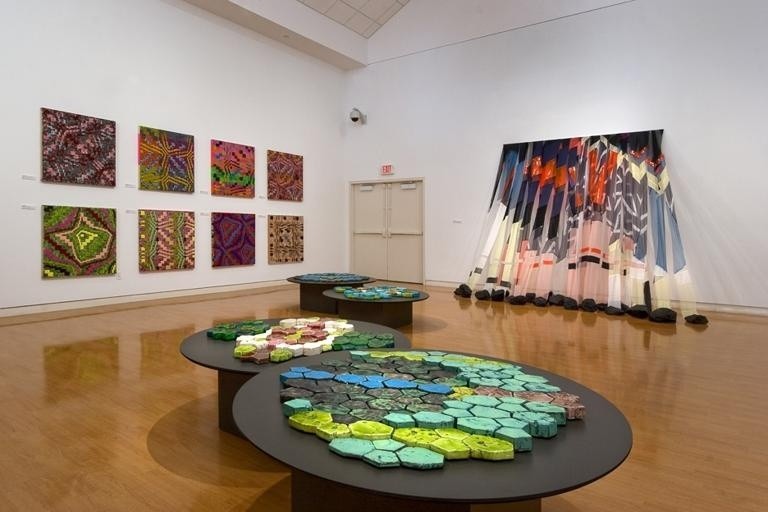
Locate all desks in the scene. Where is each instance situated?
[178,317,413,439]
[286,273,380,313]
[230,348,632,512]
[320,285,430,326]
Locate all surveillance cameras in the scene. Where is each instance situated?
[350,111,360,121]
